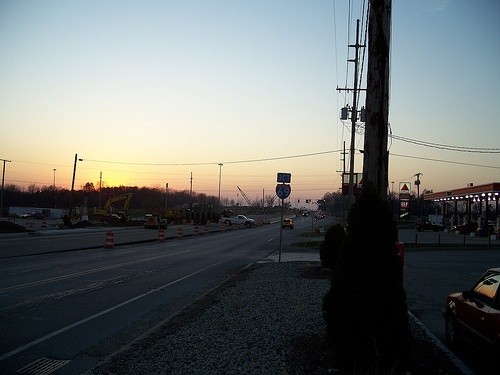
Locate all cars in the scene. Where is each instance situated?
[282,218,295,229]
[443,268,500,375]
[300,209,310,217]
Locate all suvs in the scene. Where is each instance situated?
[416,220,443,232]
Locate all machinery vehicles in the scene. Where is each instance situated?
[94,192,132,221]
[236,185,262,214]
[266,193,291,214]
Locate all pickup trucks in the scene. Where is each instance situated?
[221,215,255,228]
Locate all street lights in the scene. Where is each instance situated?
[53,168,57,190]
[218,163,223,206]
